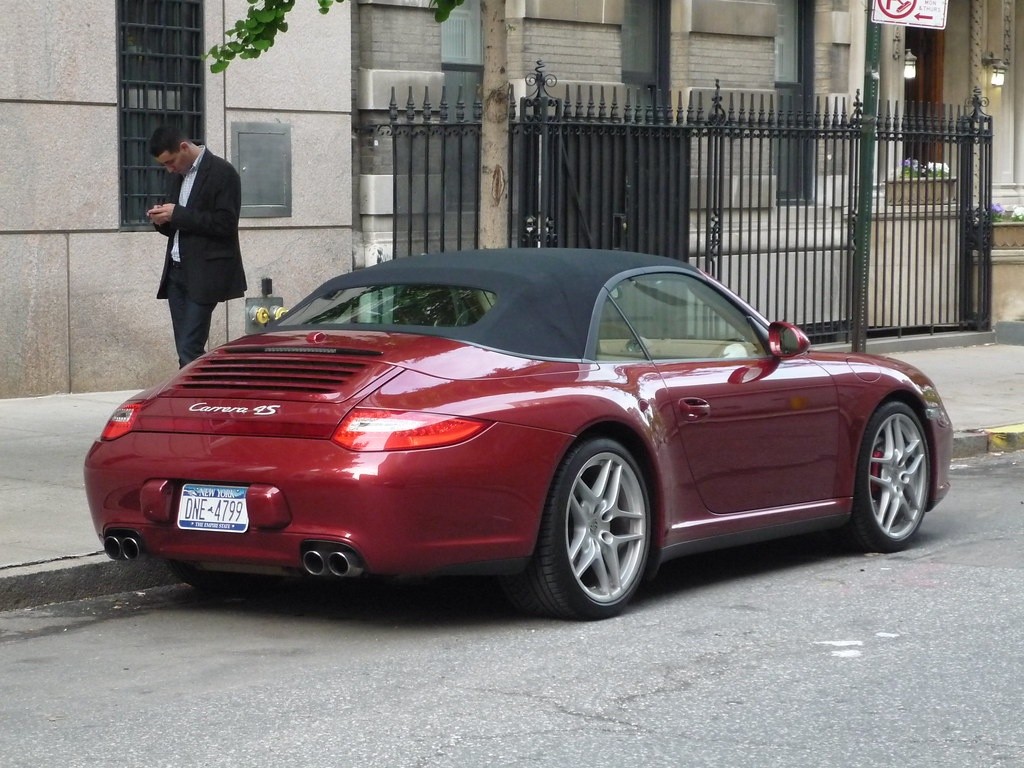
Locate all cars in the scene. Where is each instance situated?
[82,244,953,622]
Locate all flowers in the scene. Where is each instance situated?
[991,203,1024,222]
[900,159,949,178]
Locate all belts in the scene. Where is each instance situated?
[172,261,181,267]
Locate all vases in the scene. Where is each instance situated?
[991,222,1024,250]
[884,178,957,205]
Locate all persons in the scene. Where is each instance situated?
[146,127,248,371]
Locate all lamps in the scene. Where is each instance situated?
[903,49,917,79]
[983,52,1007,87]
